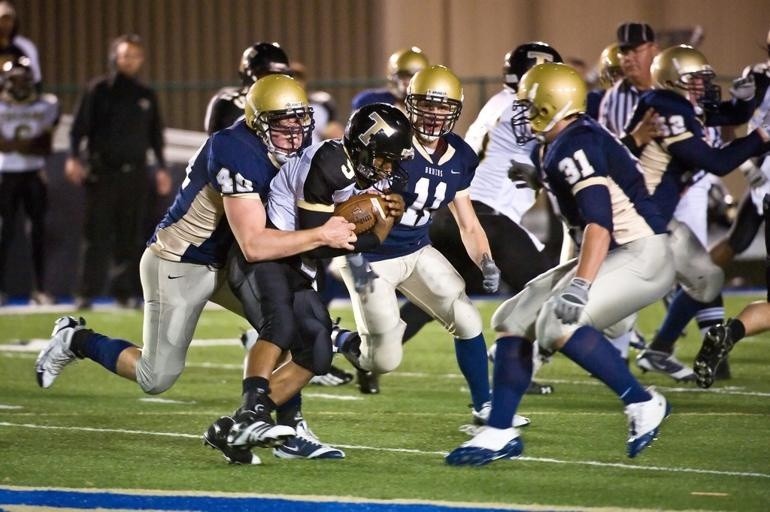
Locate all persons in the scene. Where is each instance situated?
[1,1,172,307]
[34,23,770,467]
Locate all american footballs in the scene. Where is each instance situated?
[335,195,392,236]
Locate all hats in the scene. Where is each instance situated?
[615,22,656,54]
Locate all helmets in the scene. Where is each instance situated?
[244,71,314,144]
[342,101,413,169]
[502,40,563,93]
[595,42,623,92]
[517,62,589,134]
[649,44,713,101]
[236,41,295,88]
[386,46,430,82]
[403,63,465,118]
[1,54,33,87]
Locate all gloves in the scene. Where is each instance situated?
[760,108,770,140]
[727,65,757,102]
[506,158,539,191]
[479,252,500,294]
[553,275,593,326]
[743,165,768,192]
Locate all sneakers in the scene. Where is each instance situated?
[471,400,532,430]
[629,326,696,383]
[623,383,672,459]
[240,315,380,395]
[269,411,346,462]
[446,426,523,469]
[35,313,87,389]
[226,408,297,451]
[692,322,735,390]
[203,415,264,467]
[487,343,555,395]
